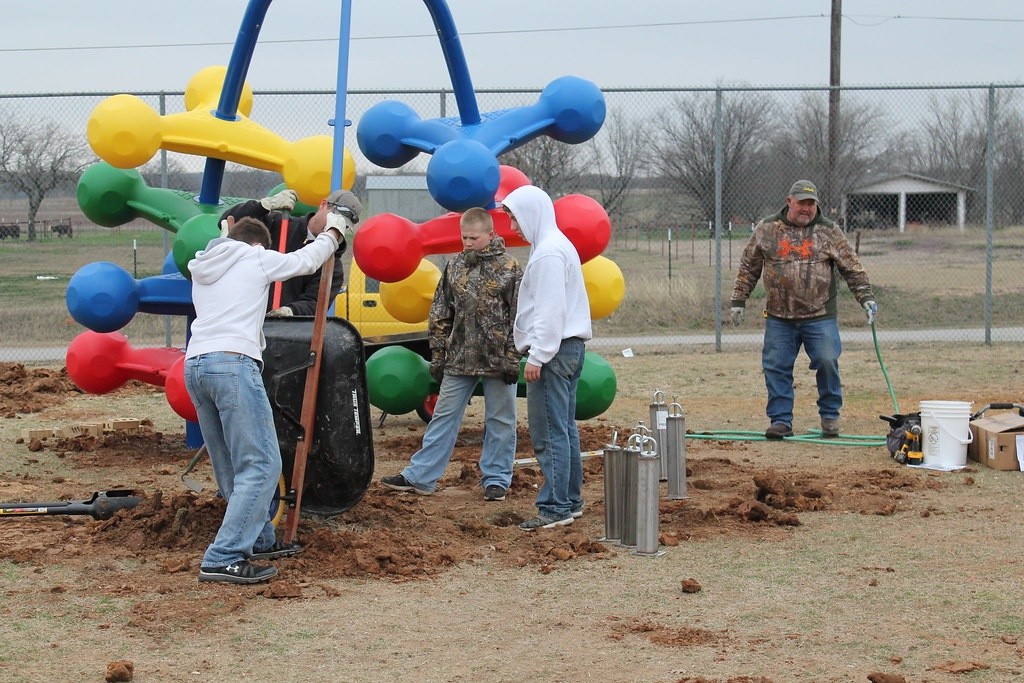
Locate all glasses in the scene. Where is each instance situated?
[328,201,359,223]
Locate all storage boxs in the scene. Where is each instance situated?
[969,411,1024,471]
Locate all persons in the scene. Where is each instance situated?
[184,212,346,583]
[729,179,878,441]
[217,189,361,318]
[381,206,523,501]
[501,184,592,531]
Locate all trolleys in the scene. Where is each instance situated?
[225,214,376,517]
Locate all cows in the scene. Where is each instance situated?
[48,225,70,239]
[0,226,20,240]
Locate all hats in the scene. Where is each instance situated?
[326,189,362,233]
[789,180,819,202]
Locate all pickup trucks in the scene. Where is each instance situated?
[331,259,444,338]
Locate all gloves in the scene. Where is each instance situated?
[430,348,445,380]
[731,307,744,328]
[863,301,878,324]
[260,190,297,211]
[499,356,519,385]
[324,211,346,238]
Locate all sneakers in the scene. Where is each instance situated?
[483,483,506,501]
[381,472,433,496]
[764,423,794,439]
[198,559,278,584]
[821,419,840,436]
[254,542,302,559]
[574,512,583,517]
[518,513,574,530]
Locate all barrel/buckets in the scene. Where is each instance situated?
[918,400,974,467]
